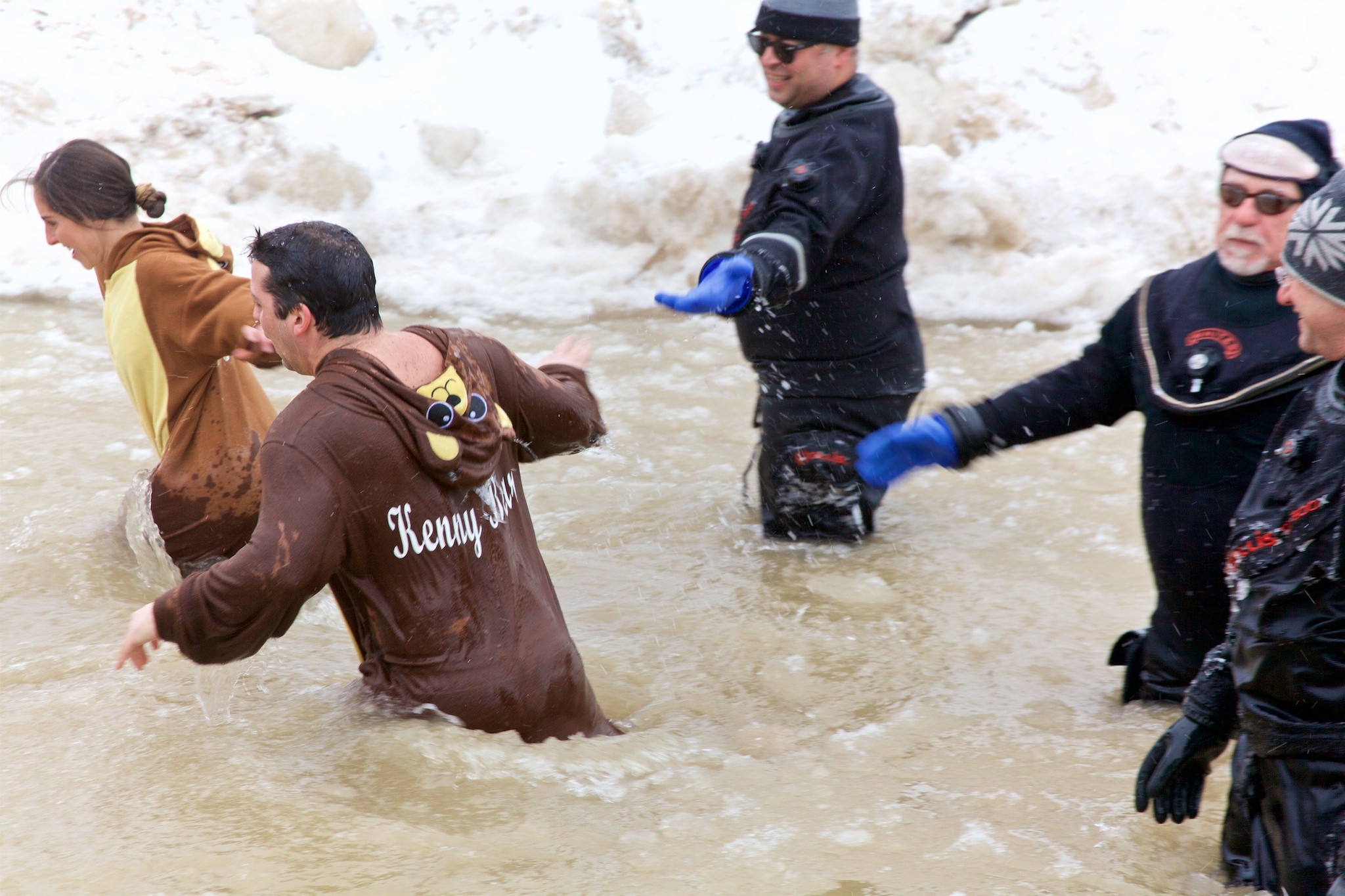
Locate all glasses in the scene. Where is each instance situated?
[748,27,822,63]
[1275,266,1297,288]
[1219,183,1305,216]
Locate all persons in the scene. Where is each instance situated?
[0,138,280,583]
[1135,168,1345,896]
[113,221,624,742]
[648,0,926,544]
[855,118,1345,706]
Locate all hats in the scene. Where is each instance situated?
[754,0,860,47]
[1279,168,1345,306]
[1220,134,1319,179]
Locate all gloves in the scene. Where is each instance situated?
[855,401,989,489]
[1135,715,1231,825]
[654,254,753,314]
